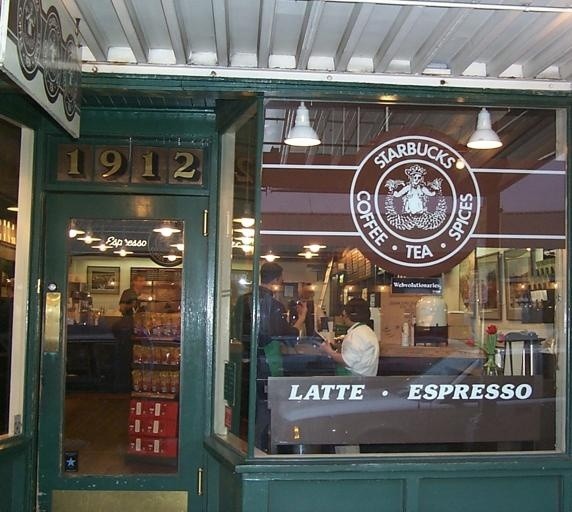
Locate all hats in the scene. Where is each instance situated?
[345,298,370,322]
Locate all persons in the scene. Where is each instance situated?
[321,295,380,455]
[234,263,308,454]
[113,274,146,394]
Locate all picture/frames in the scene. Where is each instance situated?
[87,266,120,295]
[458,247,533,320]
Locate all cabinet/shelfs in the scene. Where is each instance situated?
[125,335,179,469]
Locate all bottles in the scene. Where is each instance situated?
[512,265,555,309]
[0,219,16,245]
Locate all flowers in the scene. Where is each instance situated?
[465,323,504,353]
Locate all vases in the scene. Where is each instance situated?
[480,354,500,376]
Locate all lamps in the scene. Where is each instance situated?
[152,220,182,239]
[284,101,321,146]
[240,121,256,228]
[466,107,503,150]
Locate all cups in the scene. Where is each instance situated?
[401,322,411,348]
